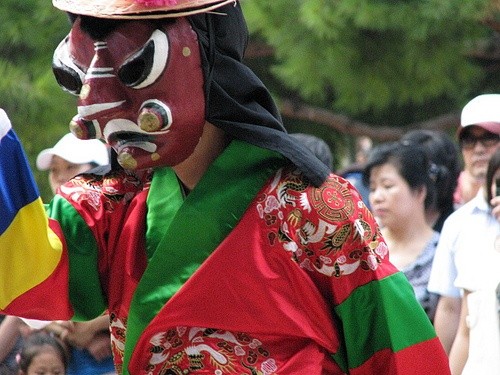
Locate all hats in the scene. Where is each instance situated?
[36,133,109,170]
[460,94,500,135]
[51,0,236,19]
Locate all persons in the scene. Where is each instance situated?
[0,0,452,374]
[287,129,480,234]
[0,132,117,375]
[364,141,441,325]
[449,146,500,375]
[425,94,500,357]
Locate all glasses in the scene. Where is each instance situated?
[462,132,500,150]
